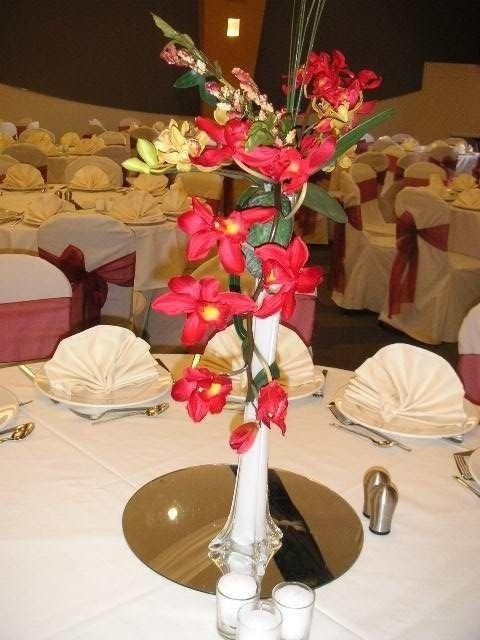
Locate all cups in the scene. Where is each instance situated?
[214,571,261,640]
[235,600,283,640]
[272,581,316,640]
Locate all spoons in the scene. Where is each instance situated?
[1,422,34,444]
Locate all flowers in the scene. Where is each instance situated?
[122,0,394,453]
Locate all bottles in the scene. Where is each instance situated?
[362,467,398,536]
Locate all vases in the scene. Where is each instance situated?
[208,278,284,570]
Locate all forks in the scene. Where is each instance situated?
[453,453,474,482]
[314,370,328,401]
[328,403,412,454]
[67,402,169,426]
[330,423,400,447]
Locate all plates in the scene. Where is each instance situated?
[33,363,172,406]
[431,185,480,212]
[1,177,187,226]
[228,369,325,407]
[335,382,479,440]
[469,446,480,482]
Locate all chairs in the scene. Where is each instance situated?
[1,117,480,404]
